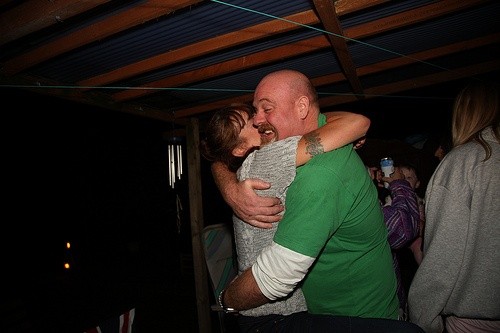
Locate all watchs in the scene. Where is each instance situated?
[217,288,240,313]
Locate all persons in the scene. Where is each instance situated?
[368,79,500,333]
[199,69,404,321]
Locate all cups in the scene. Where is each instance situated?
[380,156,395,189]
